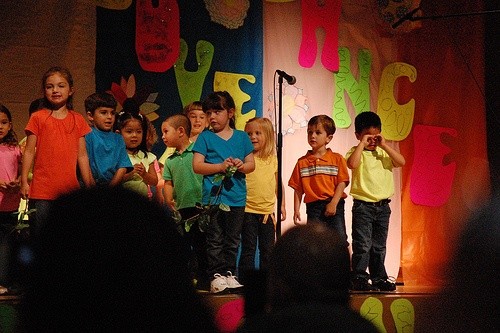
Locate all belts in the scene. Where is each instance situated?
[374,199,392,207]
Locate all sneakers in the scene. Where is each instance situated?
[210,272,233,295]
[226,270,244,292]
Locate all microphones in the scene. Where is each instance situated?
[392,7,418,29]
[276,70,296,85]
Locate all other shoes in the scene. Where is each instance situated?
[351,276,378,291]
[372,279,396,291]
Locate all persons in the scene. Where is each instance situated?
[0,103,21,231]
[236,225,381,333]
[239,117,287,272]
[115,110,158,194]
[20,66,96,225]
[12,183,218,333]
[159,101,209,177]
[161,114,205,278]
[346,111,405,292]
[288,114,351,272]
[191,91,255,295]
[15,97,55,183]
[83,92,134,185]
